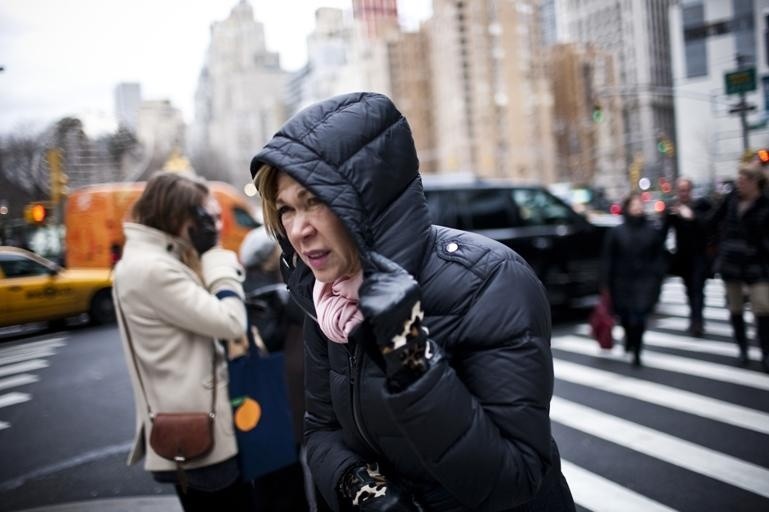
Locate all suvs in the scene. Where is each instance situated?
[418,176,672,332]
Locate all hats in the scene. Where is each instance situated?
[237,222,283,270]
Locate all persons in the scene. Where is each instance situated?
[113,172,255,509]
[661,177,716,337]
[600,191,670,374]
[239,225,288,295]
[713,161,767,369]
[248,91,575,509]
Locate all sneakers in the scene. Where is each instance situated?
[684,322,707,339]
[622,335,644,369]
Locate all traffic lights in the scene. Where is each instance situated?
[23,200,54,225]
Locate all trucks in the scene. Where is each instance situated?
[58,177,267,271]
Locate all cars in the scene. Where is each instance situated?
[0,243,118,332]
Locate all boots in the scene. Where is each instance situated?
[730,311,750,366]
[755,310,769,374]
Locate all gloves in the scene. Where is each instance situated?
[189,205,218,253]
[356,246,435,383]
[347,464,424,512]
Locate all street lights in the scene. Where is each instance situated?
[593,104,602,121]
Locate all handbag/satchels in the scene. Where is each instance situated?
[591,294,615,350]
[149,410,216,462]
[217,324,299,482]
